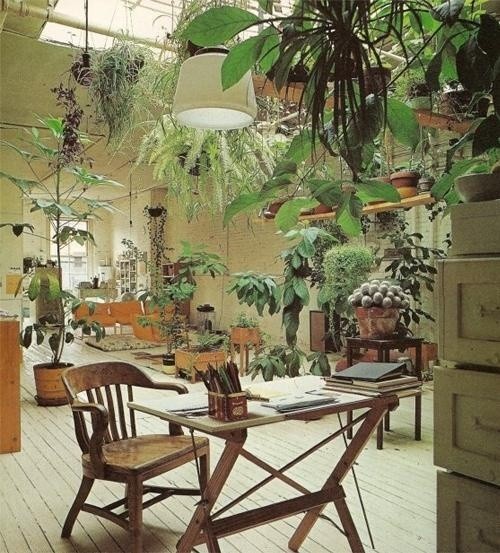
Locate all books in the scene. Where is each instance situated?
[165,361,423,417]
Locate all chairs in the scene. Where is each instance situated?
[59,361,210,553]
[74,300,144,335]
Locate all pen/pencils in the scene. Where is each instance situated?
[193,361,241,393]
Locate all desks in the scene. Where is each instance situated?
[346,337,425,449]
[126,379,421,552]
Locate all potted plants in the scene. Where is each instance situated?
[1,109,128,407]
[347,279,410,340]
[49,0,500,223]
[142,201,174,276]
[135,281,260,382]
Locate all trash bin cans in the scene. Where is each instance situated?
[23,307,30,318]
